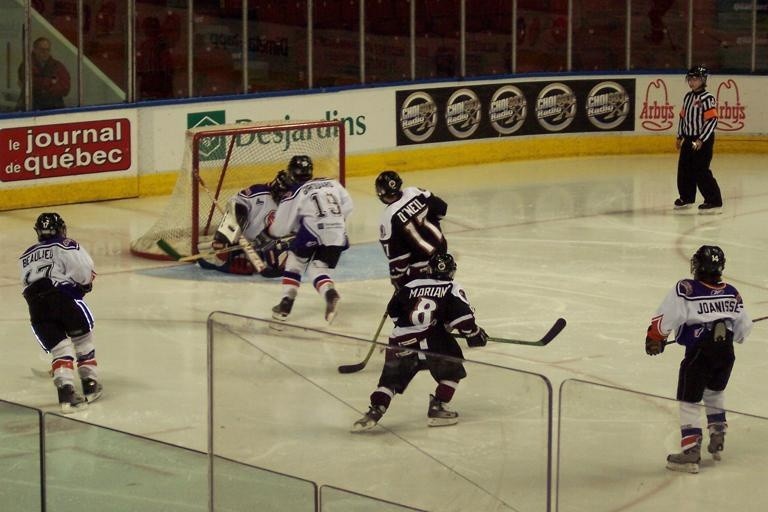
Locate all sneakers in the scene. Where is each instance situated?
[325,289,340,321]
[82,378,102,395]
[667,448,701,464]
[428,394,458,418]
[708,433,724,453]
[675,199,695,206]
[272,297,294,315]
[58,384,84,406]
[354,405,386,427]
[699,203,722,209]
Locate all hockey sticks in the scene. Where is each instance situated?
[156,235,295,261]
[338,287,397,373]
[30,365,55,377]
[451,318,567,347]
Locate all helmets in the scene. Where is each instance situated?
[426,253,456,281]
[34,213,67,241]
[686,65,708,80]
[691,246,726,275]
[277,170,300,189]
[288,156,312,181]
[375,171,402,204]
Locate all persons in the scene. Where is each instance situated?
[671,65,723,209]
[352,253,492,430]
[642,244,755,465]
[212,169,298,278]
[369,169,451,290]
[14,36,73,112]
[18,212,105,405]
[269,154,355,317]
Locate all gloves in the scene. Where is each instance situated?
[465,328,488,347]
[646,336,664,356]
